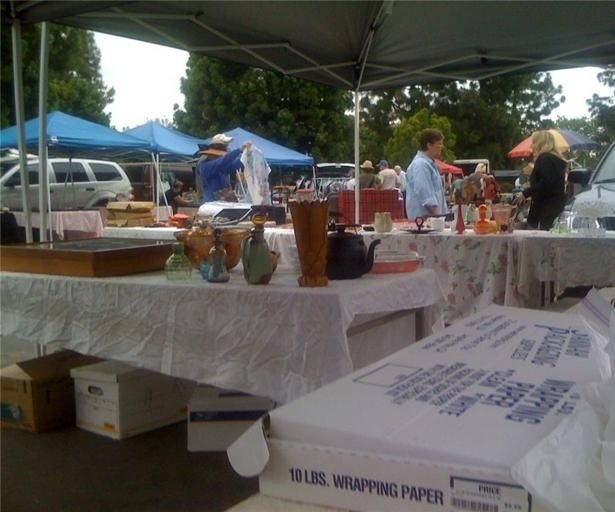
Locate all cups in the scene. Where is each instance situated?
[552,199,610,235]
[294,187,315,203]
[427,216,445,232]
[373,211,392,233]
[199,260,212,280]
[492,200,518,235]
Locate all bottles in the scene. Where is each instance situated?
[453,197,491,235]
[164,241,193,283]
[208,228,230,282]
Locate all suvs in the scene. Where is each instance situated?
[0,157,136,212]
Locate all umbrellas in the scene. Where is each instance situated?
[507,127,601,158]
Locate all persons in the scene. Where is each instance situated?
[566,167,575,195]
[195,133,245,200]
[405,128,450,220]
[158,180,194,214]
[393,164,408,220]
[346,159,402,191]
[512,130,567,231]
[199,139,253,203]
[450,161,501,205]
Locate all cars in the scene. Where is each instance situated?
[550,137,615,236]
[314,162,355,198]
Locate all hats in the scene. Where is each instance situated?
[197,140,228,157]
[376,160,389,168]
[359,160,374,169]
[212,134,233,143]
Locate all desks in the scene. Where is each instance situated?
[0,209,99,241]
[0,263,434,404]
[104,226,615,309]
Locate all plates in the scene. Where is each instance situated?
[372,259,420,274]
[403,227,431,234]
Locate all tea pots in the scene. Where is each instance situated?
[241,214,277,286]
[326,223,381,281]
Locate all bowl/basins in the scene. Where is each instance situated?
[173,228,248,272]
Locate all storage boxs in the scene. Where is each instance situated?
[188,383,276,452]
[228,304,600,512]
[0,350,108,434]
[70,359,197,440]
